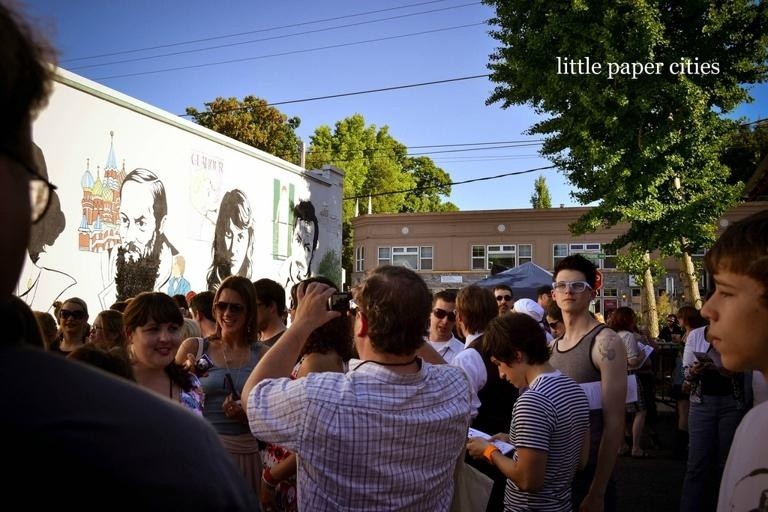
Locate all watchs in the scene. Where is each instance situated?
[483,443,501,465]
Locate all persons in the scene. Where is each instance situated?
[658,314,683,379]
[671,305,710,434]
[493,284,513,317]
[423,290,466,363]
[51,299,90,344]
[250,279,289,345]
[239,264,472,512]
[46,297,90,356]
[542,304,566,336]
[88,308,128,358]
[173,273,271,512]
[33,312,73,359]
[173,294,202,346]
[187,290,216,338]
[682,326,744,512]
[546,253,627,512]
[96,292,206,416]
[702,208,768,512]
[535,285,554,308]
[450,286,520,512]
[0,0,260,512]
[259,277,353,512]
[606,307,651,459]
[509,298,554,347]
[467,313,590,512]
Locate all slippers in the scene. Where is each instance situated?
[629,446,651,460]
[617,443,632,456]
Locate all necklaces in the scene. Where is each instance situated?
[217,336,242,366]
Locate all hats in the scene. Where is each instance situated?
[513,298,545,322]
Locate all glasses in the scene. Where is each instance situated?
[253,302,267,309]
[495,295,514,302]
[429,307,457,322]
[213,301,245,314]
[551,280,598,294]
[56,308,86,321]
[1,154,57,229]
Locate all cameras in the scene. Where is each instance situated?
[195,355,213,377]
[331,293,353,311]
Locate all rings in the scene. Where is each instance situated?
[225,406,234,412]
